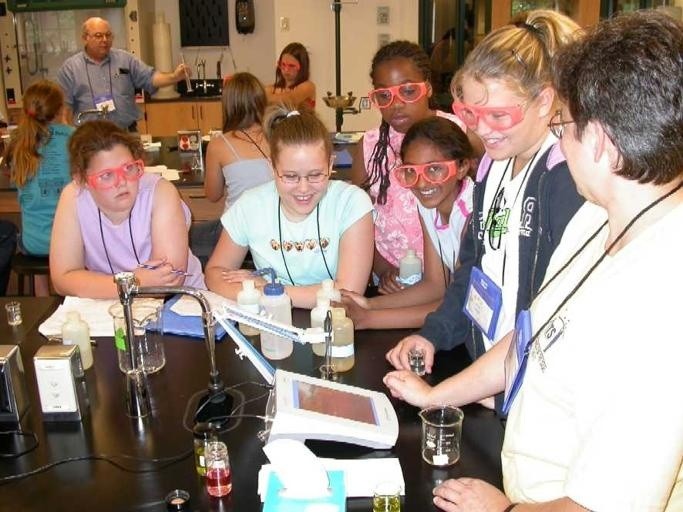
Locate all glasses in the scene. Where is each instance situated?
[367,81,427,109]
[272,154,331,184]
[86,158,144,191]
[547,112,577,139]
[85,32,113,43]
[278,61,300,71]
[392,160,458,188]
[451,89,538,131]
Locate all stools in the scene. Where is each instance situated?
[11,249,50,296]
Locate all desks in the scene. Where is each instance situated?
[0,293,506,510]
[0,133,370,229]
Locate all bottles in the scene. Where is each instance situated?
[310,278,355,374]
[253,266,295,360]
[58,310,94,372]
[191,422,233,499]
[234,278,261,337]
[396,248,422,286]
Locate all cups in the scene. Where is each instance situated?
[107,298,167,374]
[406,348,426,376]
[3,300,22,327]
[416,403,463,470]
[318,364,342,384]
[370,477,401,512]
[164,488,191,512]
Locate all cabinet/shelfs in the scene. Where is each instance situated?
[143,98,225,138]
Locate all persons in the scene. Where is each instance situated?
[350,37,486,296]
[379,6,683,512]
[385,7,588,376]
[329,115,478,332]
[428,26,470,86]
[189,71,277,261]
[55,15,190,134]
[48,119,209,297]
[0,217,19,297]
[263,43,317,115]
[202,100,375,313]
[2,80,81,298]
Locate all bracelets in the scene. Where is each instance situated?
[503,501,520,512]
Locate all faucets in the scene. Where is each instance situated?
[197,63,207,90]
[112,269,227,405]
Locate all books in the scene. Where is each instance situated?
[143,290,236,341]
